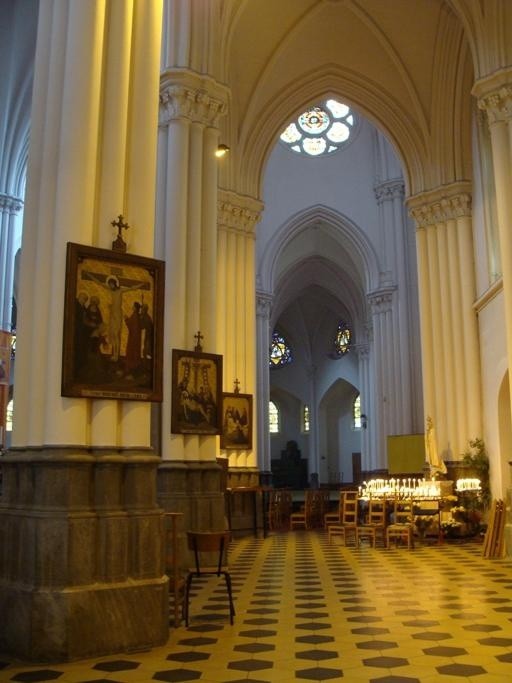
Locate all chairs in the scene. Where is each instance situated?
[324,487,415,549]
[266,487,328,531]
[185,531,235,627]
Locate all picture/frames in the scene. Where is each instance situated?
[220,393,252,450]
[61,242,165,401]
[172,350,223,434]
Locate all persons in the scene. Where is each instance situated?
[123,302,142,371]
[0,247,21,456]
[72,291,89,371]
[85,295,103,337]
[224,405,249,443]
[175,379,216,425]
[138,304,153,373]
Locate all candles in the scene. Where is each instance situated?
[358,477,438,496]
